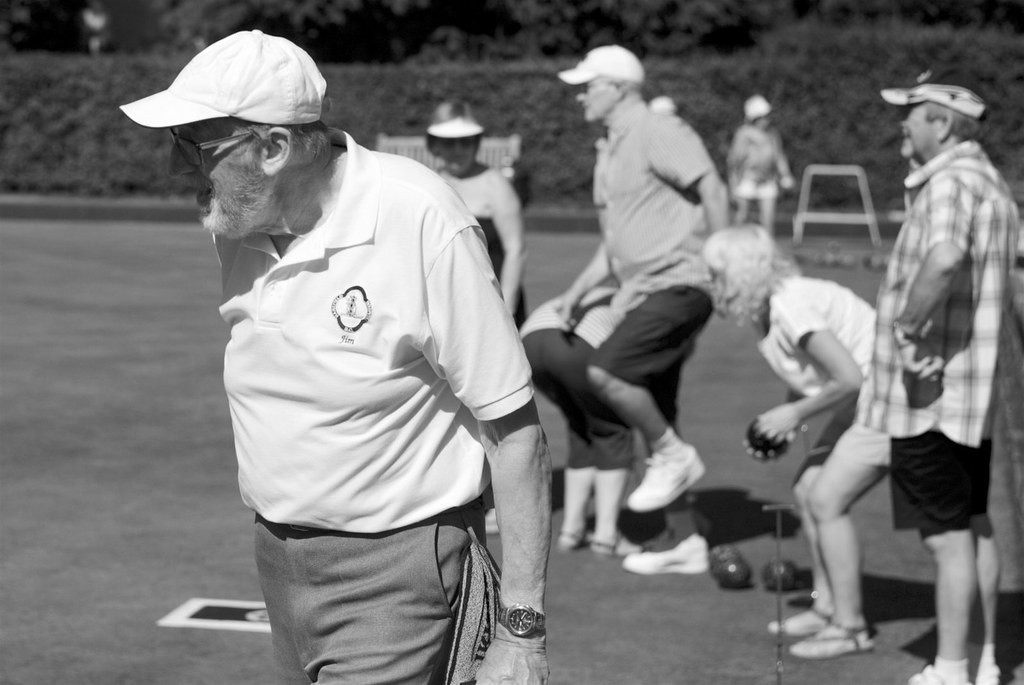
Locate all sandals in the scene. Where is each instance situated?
[789,623,874,661]
[557,525,647,558]
[767,607,834,638]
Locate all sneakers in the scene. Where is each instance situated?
[624,535,709,574]
[627,440,705,512]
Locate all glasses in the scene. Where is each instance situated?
[167,127,260,171]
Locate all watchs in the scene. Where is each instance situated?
[497,603,547,637]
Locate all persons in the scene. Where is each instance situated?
[723,94,797,242]
[118,30,555,685]
[557,43,731,576]
[703,220,910,660]
[517,254,643,558]
[422,100,530,334]
[879,59,1024,685]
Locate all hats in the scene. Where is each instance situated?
[426,117,486,139]
[743,93,771,122]
[119,29,329,129]
[556,45,645,86]
[882,81,989,121]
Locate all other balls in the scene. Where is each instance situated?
[747,419,787,460]
[708,549,751,588]
[764,560,798,590]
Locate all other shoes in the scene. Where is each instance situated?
[907,661,1002,685]
